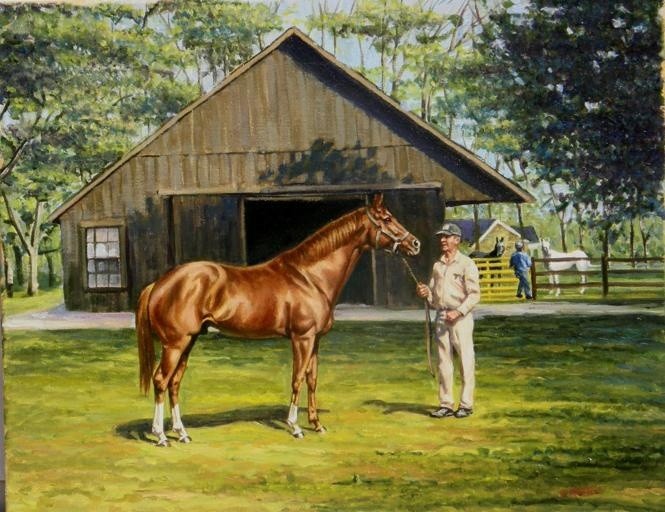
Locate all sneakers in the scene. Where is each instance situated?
[516,294,522,297]
[454,408,473,417]
[431,407,453,418]
[525,295,532,299]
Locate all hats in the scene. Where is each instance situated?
[515,240,523,249]
[435,221,462,237]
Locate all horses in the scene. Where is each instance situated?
[541,237,591,296]
[467,236,505,288]
[135,192,422,448]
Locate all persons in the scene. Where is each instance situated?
[416,221,479,418]
[508,239,535,300]
